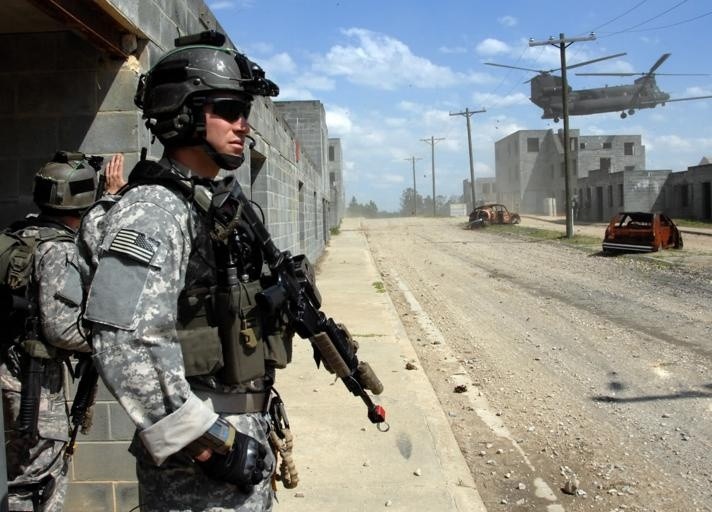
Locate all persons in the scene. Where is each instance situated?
[74,30,280,512]
[0,149,131,511]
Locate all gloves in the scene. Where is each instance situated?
[198,432,268,496]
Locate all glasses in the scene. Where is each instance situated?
[206,97,252,123]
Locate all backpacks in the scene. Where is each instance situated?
[0,223,76,366]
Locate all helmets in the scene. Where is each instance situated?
[31,150,107,215]
[133,29,278,149]
